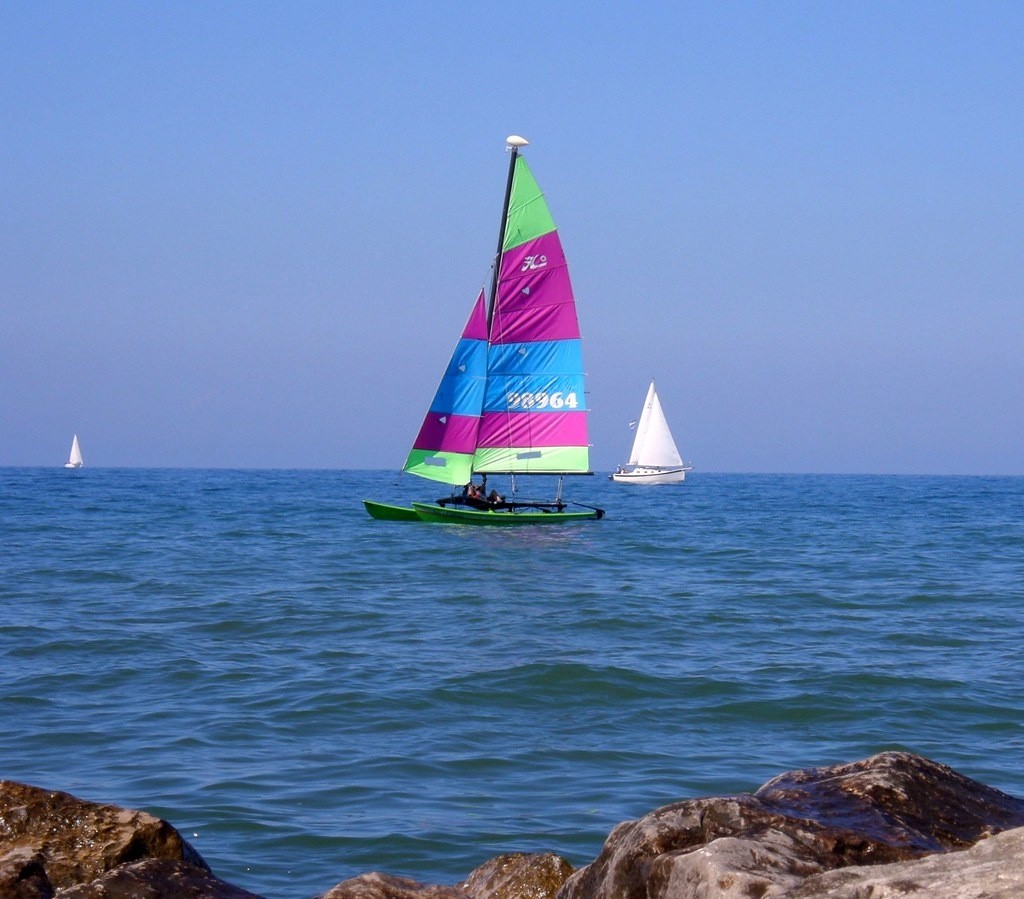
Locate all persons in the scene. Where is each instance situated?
[462,481,506,504]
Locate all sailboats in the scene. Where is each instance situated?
[64,433,83,468]
[362,135,605,524]
[613,378,693,485]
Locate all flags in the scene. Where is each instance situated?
[630,420,636,431]
